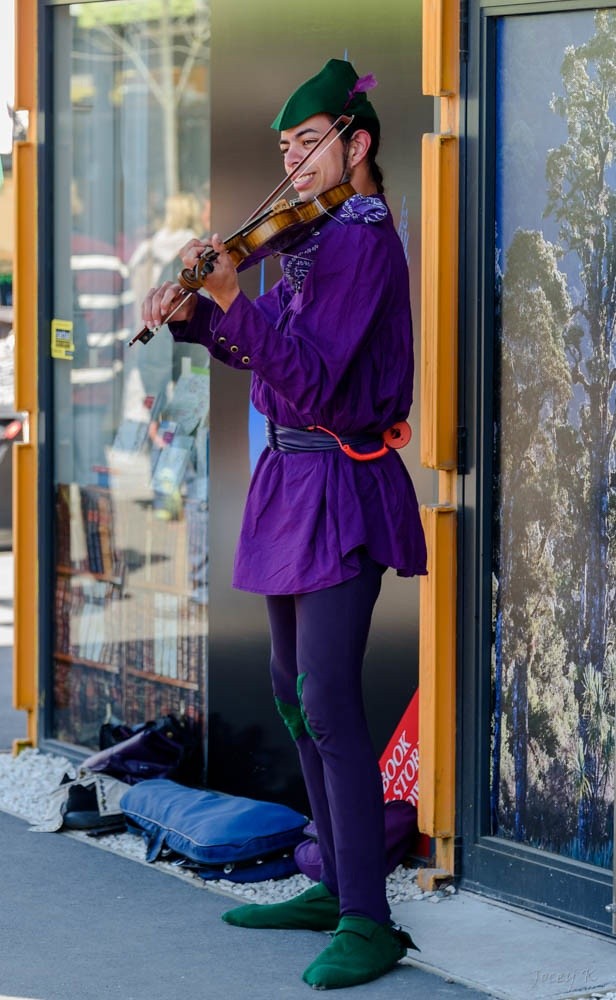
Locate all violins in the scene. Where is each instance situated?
[177,181,358,292]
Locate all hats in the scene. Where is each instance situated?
[268,58,378,133]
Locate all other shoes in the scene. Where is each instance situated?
[302,916,423,991]
[222,882,341,932]
[157,490,183,520]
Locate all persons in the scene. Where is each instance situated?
[125,192,210,519]
[50,178,133,603]
[138,57,428,988]
